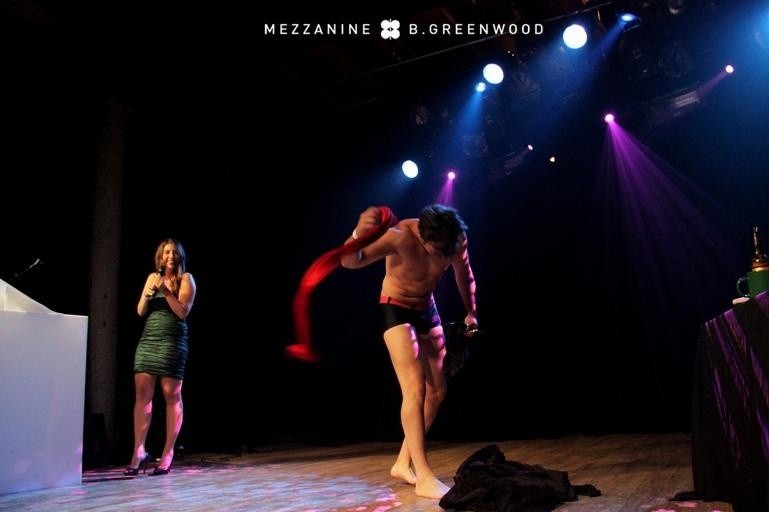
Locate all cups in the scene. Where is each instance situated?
[736,272,769,298]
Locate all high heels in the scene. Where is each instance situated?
[123,451,151,476]
[153,453,175,476]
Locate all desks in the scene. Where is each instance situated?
[686,288,769,512]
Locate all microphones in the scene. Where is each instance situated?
[150,265,166,297]
[7,257,41,282]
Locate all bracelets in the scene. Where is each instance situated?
[164,291,171,296]
[351,228,360,241]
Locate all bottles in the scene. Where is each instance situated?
[750,228,769,273]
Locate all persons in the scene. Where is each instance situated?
[123,238,197,476]
[341,203,479,500]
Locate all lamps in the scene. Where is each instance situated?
[353,0,769,183]
